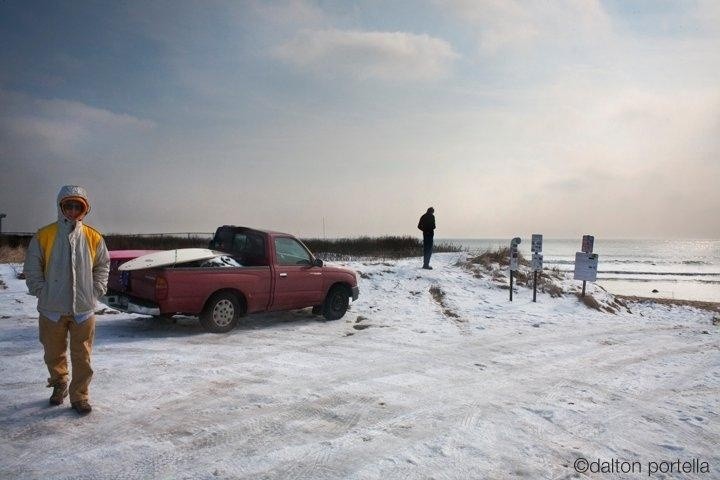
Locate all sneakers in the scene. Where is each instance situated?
[49,386,68,405]
[72,400,92,415]
[423,266,433,270]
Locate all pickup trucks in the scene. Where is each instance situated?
[97,222,360,333]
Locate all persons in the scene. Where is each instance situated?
[22,182,113,415]
[417,207,436,271]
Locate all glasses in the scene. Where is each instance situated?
[64,202,84,211]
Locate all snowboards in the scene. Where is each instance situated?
[118,249,233,271]
[108,250,166,258]
